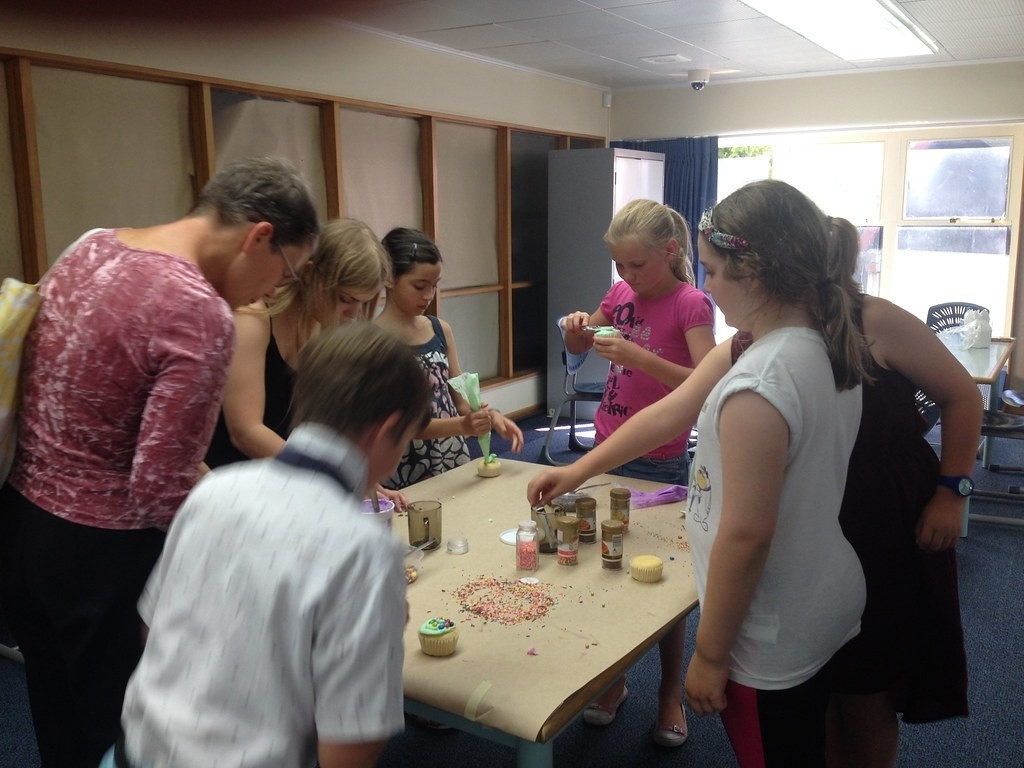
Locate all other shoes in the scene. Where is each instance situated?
[584,683,628,725]
[653,696,688,748]
[409,712,460,733]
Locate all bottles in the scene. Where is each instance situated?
[516,520,540,572]
[576,497,597,545]
[610,488,631,534]
[557,516,579,566]
[601,521,624,571]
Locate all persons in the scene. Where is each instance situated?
[562,198,717,747]
[0,160,321,768]
[526,213,984,767]
[206,218,410,511]
[378,226,523,491]
[683,181,867,768]
[113,321,431,768]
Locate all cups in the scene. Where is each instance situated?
[531,504,566,553]
[403,544,424,585]
[407,501,442,552]
[360,499,395,536]
[554,490,587,512]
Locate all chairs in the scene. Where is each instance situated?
[926,303,1024,526]
[543,318,607,464]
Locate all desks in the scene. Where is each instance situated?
[389,453,699,768]
[937,333,1016,468]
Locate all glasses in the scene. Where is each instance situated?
[244,203,303,289]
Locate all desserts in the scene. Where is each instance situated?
[478,453,501,477]
[631,555,664,583]
[418,617,459,656]
[594,329,620,338]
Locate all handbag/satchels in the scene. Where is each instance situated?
[1,223,110,483]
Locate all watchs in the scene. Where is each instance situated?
[938,473,975,496]
